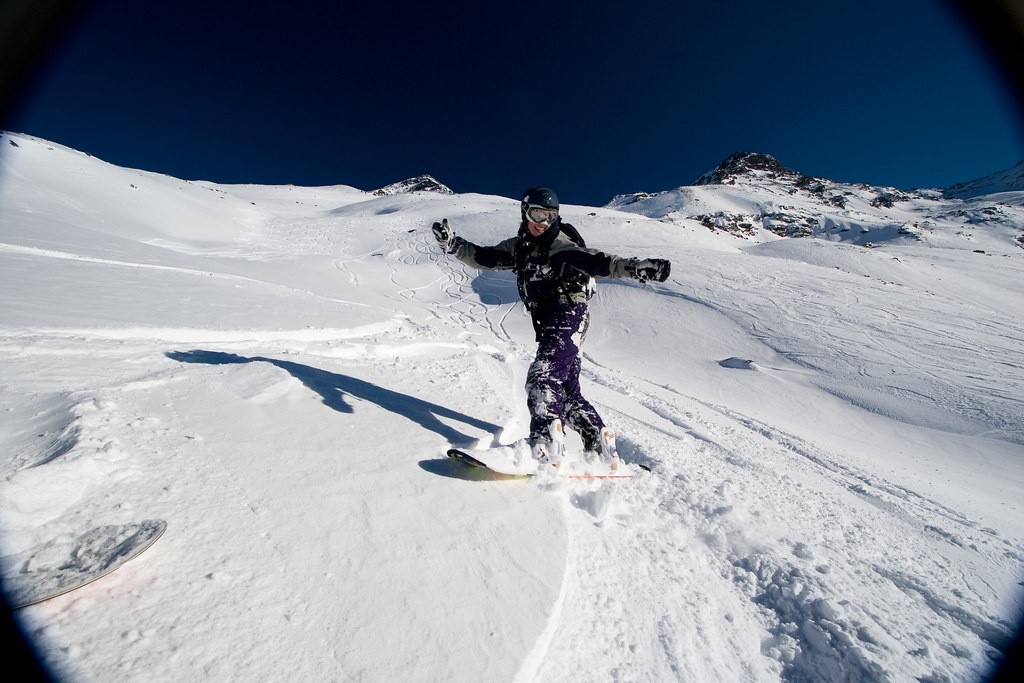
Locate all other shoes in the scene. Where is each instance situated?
[584,425,620,474]
[528,415,566,469]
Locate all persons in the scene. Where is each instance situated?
[432,187,671,464]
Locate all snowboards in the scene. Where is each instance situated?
[446,447,652,479]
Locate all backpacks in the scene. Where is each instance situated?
[513,222,597,312]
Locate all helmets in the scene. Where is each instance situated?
[521,186,560,223]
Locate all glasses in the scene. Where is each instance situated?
[519,201,559,225]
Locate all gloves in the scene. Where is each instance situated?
[627,258,671,284]
[432,219,459,256]
